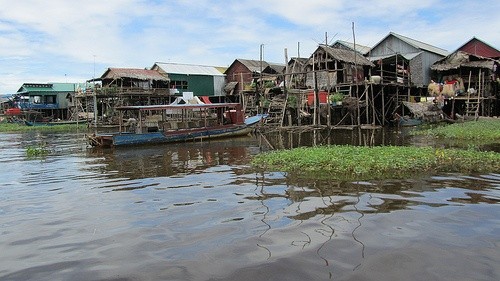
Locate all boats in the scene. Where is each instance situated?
[88,96,270,148]
